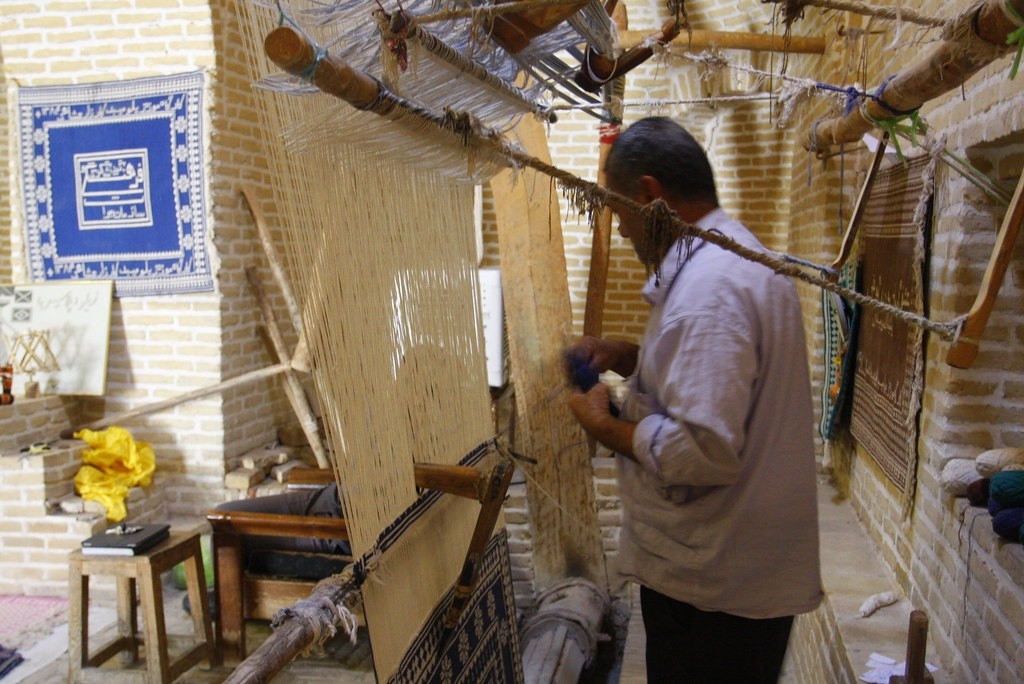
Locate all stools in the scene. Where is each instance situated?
[68,531,216,684]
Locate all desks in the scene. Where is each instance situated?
[207,489,365,663]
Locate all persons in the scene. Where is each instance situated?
[567,116,825,684]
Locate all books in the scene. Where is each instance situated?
[82,523,170,556]
